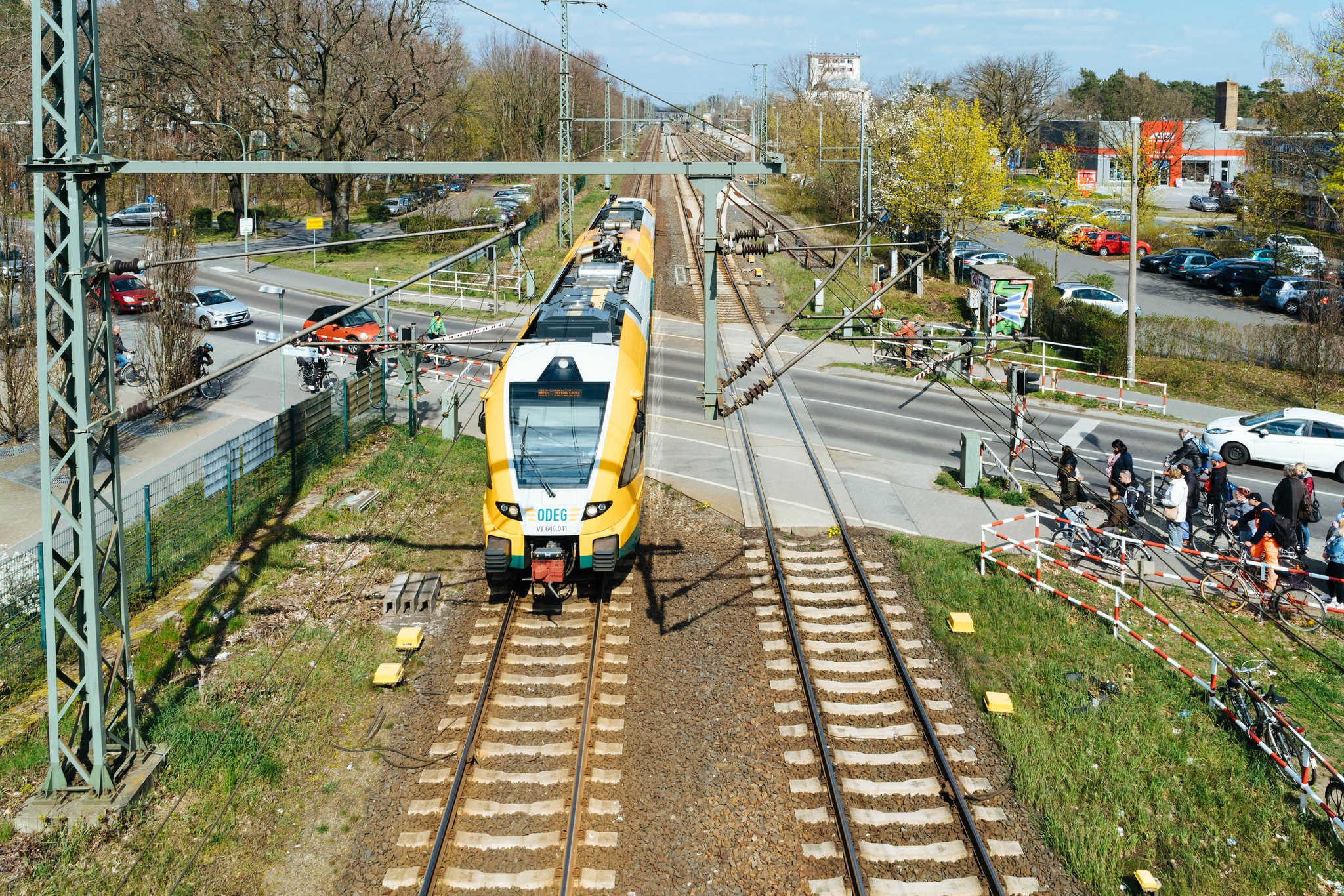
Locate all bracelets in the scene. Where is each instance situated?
[1300,425,1304,428]
[128,350,131,352]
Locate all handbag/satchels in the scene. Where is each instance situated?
[1180,521,1189,539]
[1307,499,1322,523]
[1076,484,1088,503]
[1164,505,1179,521]
[1227,481,1237,502]
[1297,493,1313,522]
[1064,506,1084,524]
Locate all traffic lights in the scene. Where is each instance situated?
[1024,367,1040,395]
[510,233,518,245]
[396,324,411,350]
[483,246,494,261]
[994,294,1008,314]
[877,264,890,283]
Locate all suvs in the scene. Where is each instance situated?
[107,202,172,227]
[1049,281,1144,324]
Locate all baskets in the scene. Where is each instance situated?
[295,357,306,364]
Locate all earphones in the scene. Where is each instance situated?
[364,350,366,351]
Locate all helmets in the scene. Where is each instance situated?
[203,343,213,351]
[434,310,441,316]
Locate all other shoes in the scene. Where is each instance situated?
[308,385,315,390]
[1182,539,1191,545]
[320,388,325,391]
[1191,508,1201,515]
[1210,533,1222,539]
[1164,548,1180,555]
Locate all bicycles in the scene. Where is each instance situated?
[169,362,222,400]
[297,358,340,396]
[89,349,149,387]
[1050,453,1328,633]
[1216,659,1344,850]
[410,333,451,367]
[335,365,388,409]
[874,328,935,372]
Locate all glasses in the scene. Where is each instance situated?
[1121,470,1129,477]
[1178,434,1181,436]
[367,349,371,351]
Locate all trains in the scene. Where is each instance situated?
[478,195,657,589]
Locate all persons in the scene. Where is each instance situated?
[1215,463,1314,594]
[1080,439,1139,555]
[1295,420,1307,435]
[356,345,379,378]
[960,321,974,371]
[299,329,332,391]
[190,342,214,397]
[1319,497,1344,610]
[122,280,139,290]
[1051,446,1079,539]
[427,311,448,359]
[1159,428,1228,555]
[113,325,132,377]
[891,314,926,370]
[207,291,218,303]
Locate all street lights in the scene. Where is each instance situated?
[258,285,287,413]
[849,87,865,280]
[813,103,822,212]
[772,106,778,183]
[189,121,250,273]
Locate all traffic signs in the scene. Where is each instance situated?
[284,345,318,359]
[256,329,281,344]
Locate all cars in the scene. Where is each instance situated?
[381,183,448,217]
[88,274,160,315]
[19,266,161,315]
[939,176,1152,257]
[458,178,548,233]
[302,305,397,353]
[1202,408,1344,483]
[161,286,250,332]
[447,178,468,192]
[852,195,1053,280]
[1139,225,1344,336]
[1209,181,1235,198]
[1189,195,1243,212]
[0,248,31,284]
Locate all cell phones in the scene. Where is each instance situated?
[1054,481,1060,486]
[1201,467,1207,473]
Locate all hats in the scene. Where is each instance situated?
[1179,459,1194,468]
[1207,453,1222,461]
[360,344,371,350]
[1244,492,1262,502]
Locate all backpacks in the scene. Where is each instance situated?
[1188,435,1213,458]
[1259,506,1297,548]
[1114,490,1148,527]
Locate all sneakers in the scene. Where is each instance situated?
[1319,594,1344,610]
[1051,529,1063,538]
[1060,534,1073,541]
[1295,543,1309,555]
[1079,546,1090,555]
